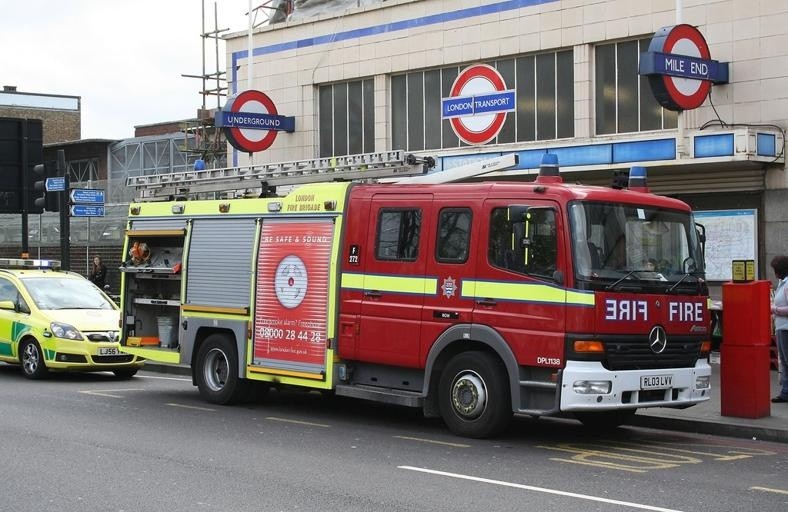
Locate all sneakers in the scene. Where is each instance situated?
[772,396,786,403]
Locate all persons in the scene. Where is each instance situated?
[766,256,788,405]
[87,254,110,292]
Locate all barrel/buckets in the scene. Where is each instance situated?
[155,315,178,350]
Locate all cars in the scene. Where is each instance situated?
[0,253,149,380]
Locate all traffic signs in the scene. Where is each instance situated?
[71,204,106,217]
[70,189,106,205]
[45,176,65,191]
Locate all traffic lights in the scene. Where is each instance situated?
[31,159,59,211]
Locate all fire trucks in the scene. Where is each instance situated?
[116,146,715,436]
